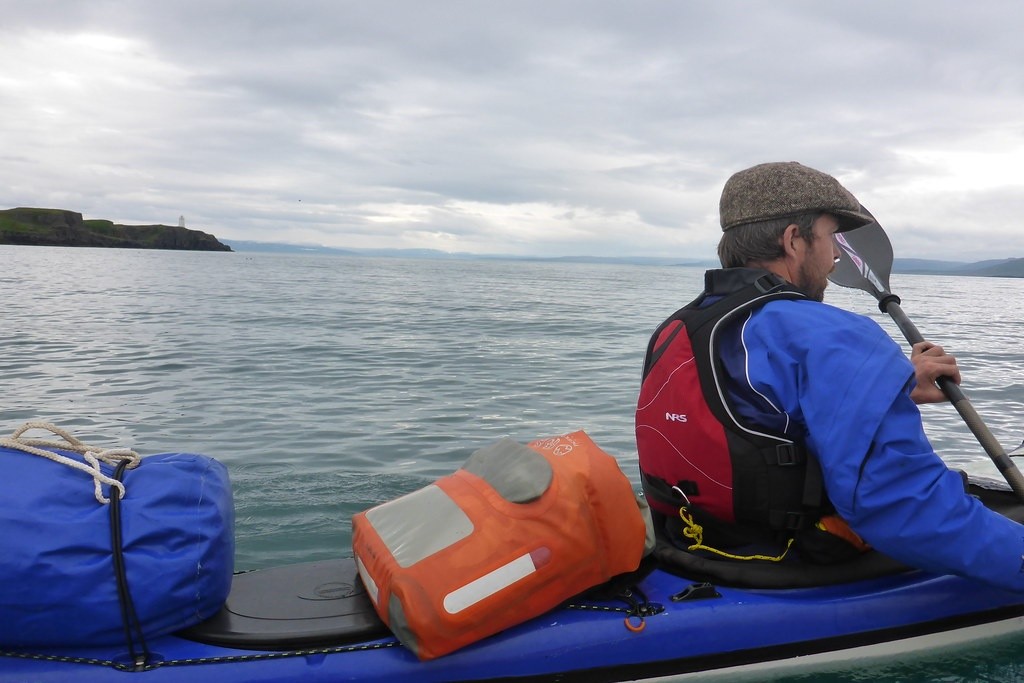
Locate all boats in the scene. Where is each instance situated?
[0,443,1024,682]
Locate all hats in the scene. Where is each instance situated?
[719,162,875,234]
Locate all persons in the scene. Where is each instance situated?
[635,159,1023,594]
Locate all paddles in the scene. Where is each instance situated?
[829,202,1024,498]
